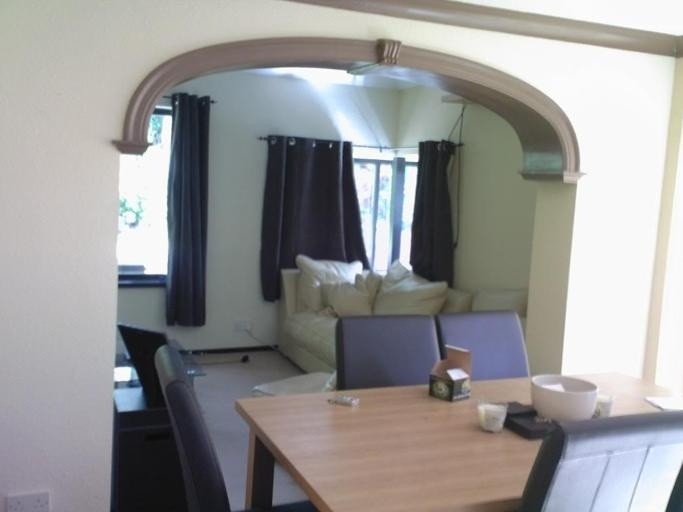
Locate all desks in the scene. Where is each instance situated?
[234,372,683,512]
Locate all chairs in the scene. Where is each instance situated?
[335,310,531,390]
[513,410,683,511]
[154,347,318,512]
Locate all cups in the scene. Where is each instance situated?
[478,404,508,431]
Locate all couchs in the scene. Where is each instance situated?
[279,269,528,374]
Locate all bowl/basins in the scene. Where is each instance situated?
[530,375,599,421]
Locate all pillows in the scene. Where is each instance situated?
[296,252,528,317]
